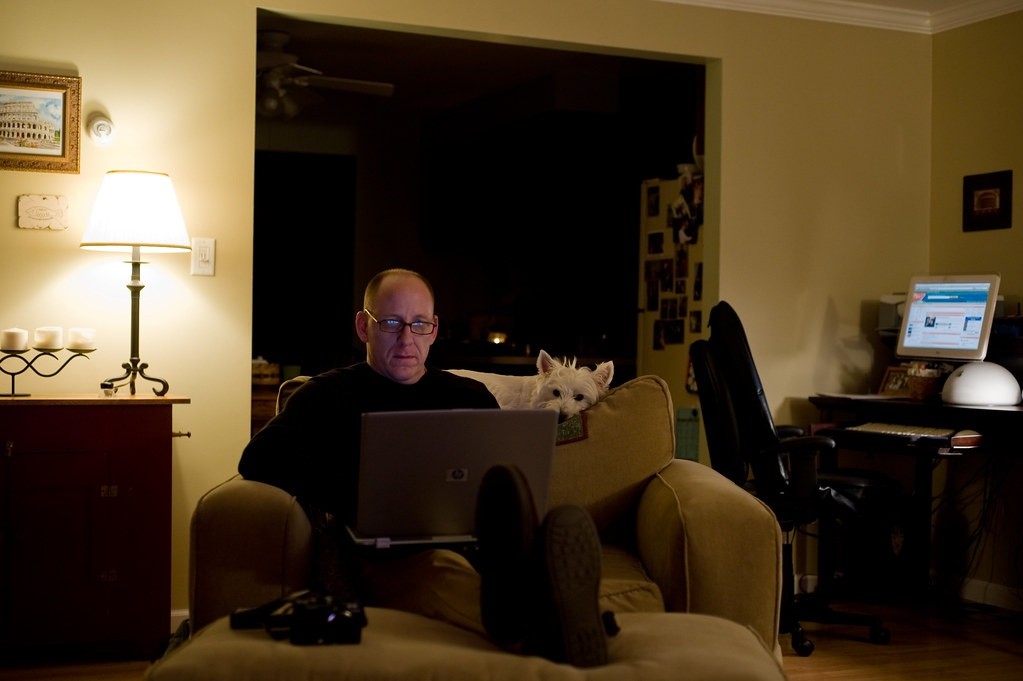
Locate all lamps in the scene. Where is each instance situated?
[79,169,192,396]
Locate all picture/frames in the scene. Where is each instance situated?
[879,365,911,393]
[962,168,1013,232]
[0,70,82,173]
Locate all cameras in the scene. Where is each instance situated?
[292,598,367,646]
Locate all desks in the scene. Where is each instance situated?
[250,387,279,438]
[807,314,1023,620]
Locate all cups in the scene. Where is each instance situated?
[909,375,935,400]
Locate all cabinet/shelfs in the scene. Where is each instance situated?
[0,393,194,673]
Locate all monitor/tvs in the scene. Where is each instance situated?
[894,274,1000,362]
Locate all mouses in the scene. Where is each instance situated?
[954,429,979,436]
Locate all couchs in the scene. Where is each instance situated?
[142,373,785,681]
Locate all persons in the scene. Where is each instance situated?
[236,269,609,669]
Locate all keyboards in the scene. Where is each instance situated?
[846,422,956,438]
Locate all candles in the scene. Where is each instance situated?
[66,326,95,349]
[0,328,28,350]
[33,326,64,348]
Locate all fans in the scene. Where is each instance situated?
[257,27,397,123]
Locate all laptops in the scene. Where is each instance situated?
[344,408,559,548]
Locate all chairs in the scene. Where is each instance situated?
[688,300,904,657]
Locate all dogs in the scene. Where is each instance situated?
[444,349,614,423]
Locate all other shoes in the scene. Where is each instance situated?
[473,462,544,652]
[541,502,605,666]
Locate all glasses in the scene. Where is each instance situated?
[364,309,436,336]
